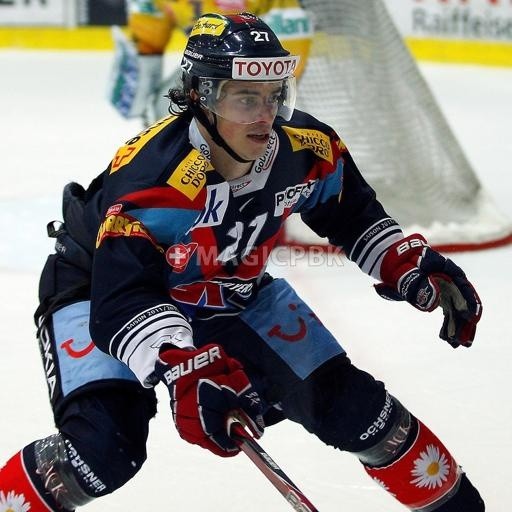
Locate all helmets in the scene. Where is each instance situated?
[179,11,301,125]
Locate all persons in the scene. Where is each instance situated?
[1,10,490,512]
[103,0,312,131]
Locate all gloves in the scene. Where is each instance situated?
[373,233,483,349]
[155,341,268,458]
